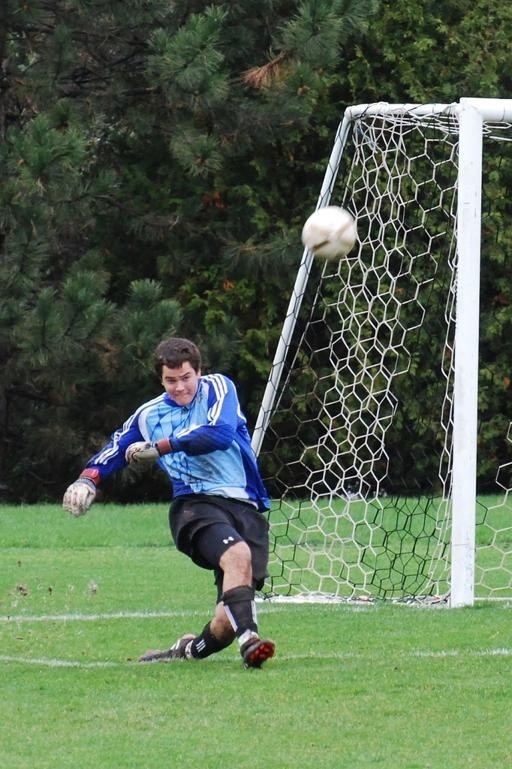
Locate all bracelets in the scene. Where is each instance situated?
[79,468,102,487]
[156,437,173,456]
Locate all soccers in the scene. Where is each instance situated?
[301,205,357,258]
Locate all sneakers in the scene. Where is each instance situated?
[136,632,197,669]
[238,634,276,670]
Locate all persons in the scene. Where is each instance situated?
[62,337,278,670]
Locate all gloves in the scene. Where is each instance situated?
[61,475,97,518]
[124,440,161,467]
